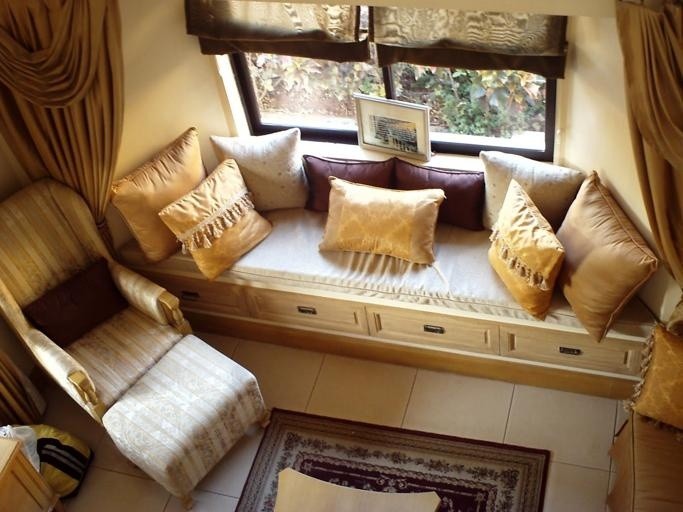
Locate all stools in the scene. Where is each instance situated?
[101,333,271,512]
[605,408,683,512]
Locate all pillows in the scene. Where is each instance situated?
[21,255,131,349]
[621,319,683,444]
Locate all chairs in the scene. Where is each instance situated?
[0,176,194,427]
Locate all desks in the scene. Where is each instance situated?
[1,437,68,512]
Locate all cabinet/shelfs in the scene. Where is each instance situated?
[244,286,372,337]
[365,304,500,355]
[497,321,645,378]
[126,266,253,319]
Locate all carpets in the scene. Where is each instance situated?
[234,405,552,512]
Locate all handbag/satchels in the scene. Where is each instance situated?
[10,422,95,499]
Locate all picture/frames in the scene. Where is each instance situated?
[352,91,432,162]
